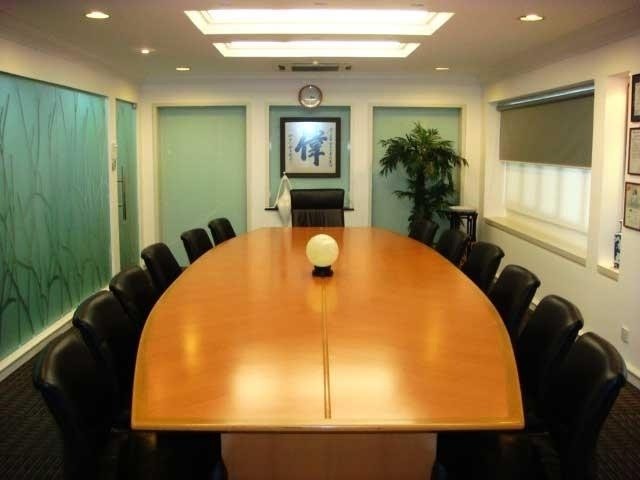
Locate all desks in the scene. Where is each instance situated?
[129,225,524,479]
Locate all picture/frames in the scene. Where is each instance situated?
[623,182,640,232]
[280,117,341,177]
[628,128,640,175]
[631,73,640,122]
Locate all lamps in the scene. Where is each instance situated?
[306,234,339,278]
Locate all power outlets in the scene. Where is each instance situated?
[620,326,630,344]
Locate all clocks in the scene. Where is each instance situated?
[298,85,323,111]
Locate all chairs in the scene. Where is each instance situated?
[291,188,344,227]
